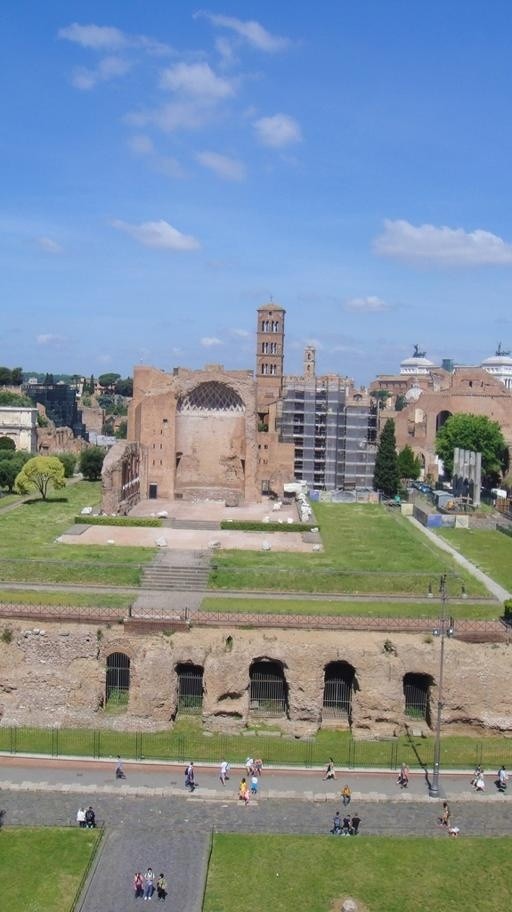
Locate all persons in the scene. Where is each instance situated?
[321,756,337,782]
[439,802,451,828]
[339,783,352,807]
[470,763,510,793]
[156,873,169,902]
[342,813,355,836]
[75,806,87,828]
[328,811,343,835]
[143,867,156,901]
[399,764,409,787]
[133,870,146,898]
[395,762,406,783]
[184,753,263,806]
[114,754,128,779]
[85,806,97,828]
[351,810,361,832]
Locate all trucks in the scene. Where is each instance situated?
[492,488,507,502]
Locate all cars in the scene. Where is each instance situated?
[412,479,434,493]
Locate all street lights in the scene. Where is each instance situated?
[426,570,467,798]
